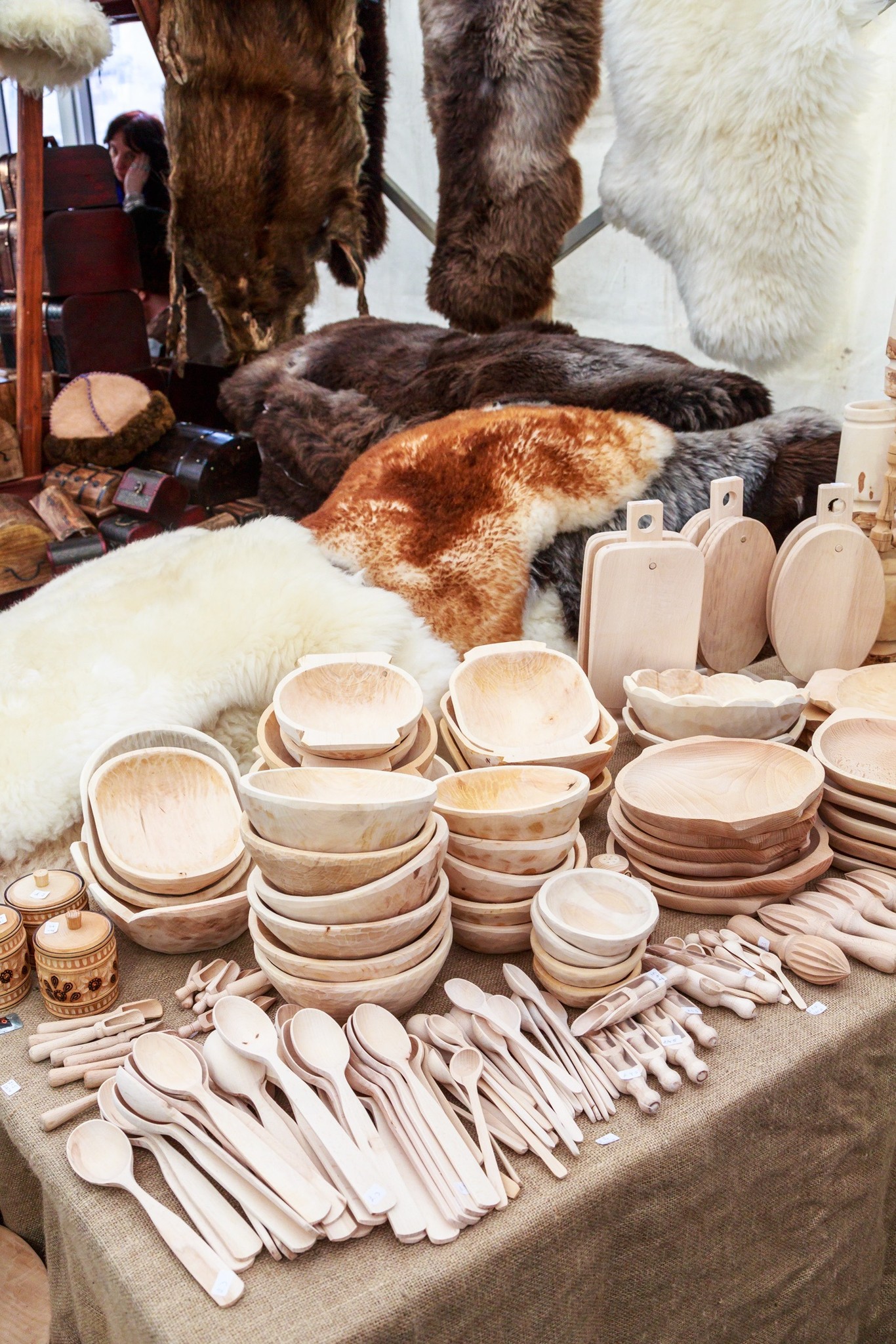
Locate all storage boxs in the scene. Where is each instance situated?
[0,206,145,298]
[0,414,266,595]
[0,288,150,378]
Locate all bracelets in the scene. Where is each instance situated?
[122,192,145,212]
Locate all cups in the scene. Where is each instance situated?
[835,399,896,513]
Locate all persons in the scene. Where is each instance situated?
[106,113,169,297]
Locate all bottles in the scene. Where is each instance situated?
[0,868,118,1021]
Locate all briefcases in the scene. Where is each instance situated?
[0,139,153,379]
[42,421,262,579]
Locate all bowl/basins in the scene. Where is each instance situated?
[67,637,896,1007]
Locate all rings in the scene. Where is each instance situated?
[131,163,138,168]
[141,164,149,170]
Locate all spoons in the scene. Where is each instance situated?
[64,961,621,1310]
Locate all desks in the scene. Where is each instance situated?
[0,735,896,1344]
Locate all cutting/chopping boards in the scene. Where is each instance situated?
[576,476,886,710]
